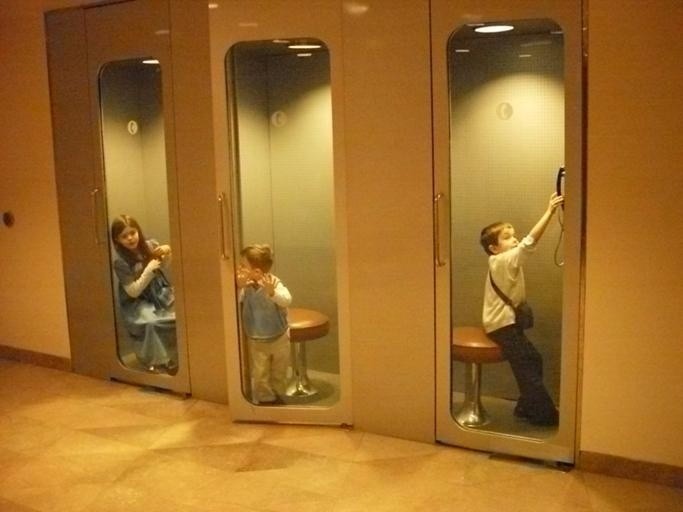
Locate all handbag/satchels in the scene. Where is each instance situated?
[152,269,176,311]
[514,305,534,331]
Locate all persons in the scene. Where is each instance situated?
[479,191,566,427]
[234,243,300,406]
[108,213,181,371]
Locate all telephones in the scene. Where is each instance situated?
[556,167,565,197]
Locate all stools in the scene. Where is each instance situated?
[280,305,335,412]
[448,324,512,430]
